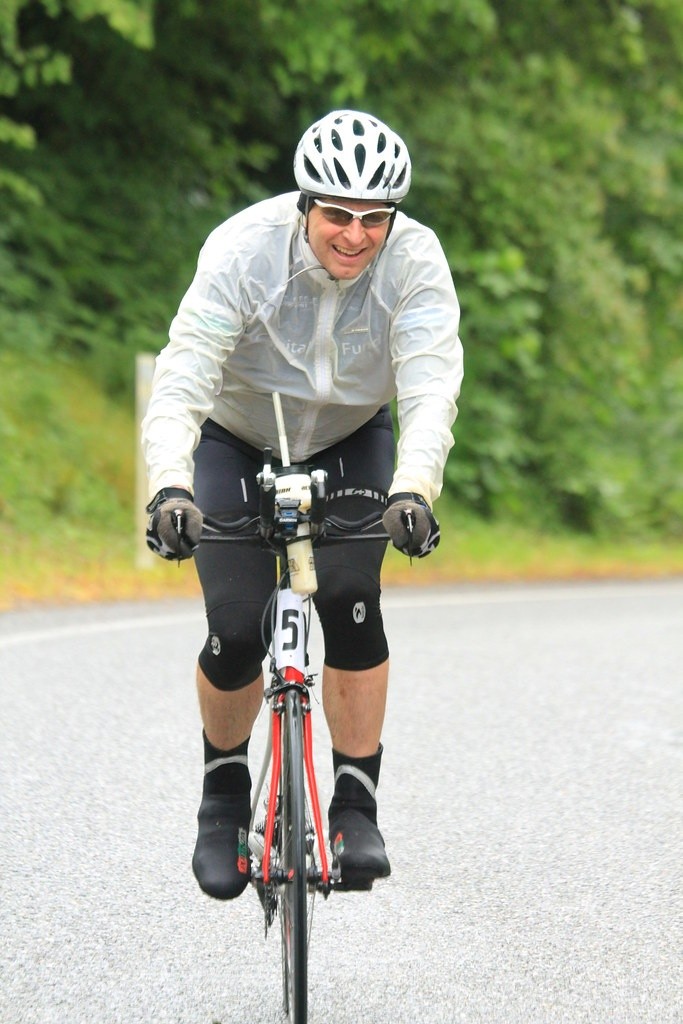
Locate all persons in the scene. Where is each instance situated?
[141,111,464,899]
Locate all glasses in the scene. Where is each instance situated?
[313,198,396,229]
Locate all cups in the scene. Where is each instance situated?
[272,464,320,594]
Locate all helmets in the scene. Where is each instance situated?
[293,109,413,205]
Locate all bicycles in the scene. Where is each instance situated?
[171,451,416,1024]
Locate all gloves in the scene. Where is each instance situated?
[144,487,203,563]
[382,494,440,559]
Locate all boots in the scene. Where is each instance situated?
[326,741,392,880]
[190,728,252,903]
